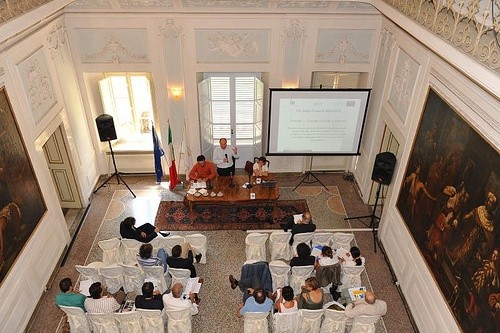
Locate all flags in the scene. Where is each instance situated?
[151,124,164,182]
[167,124,178,191]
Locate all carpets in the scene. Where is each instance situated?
[154,199,313,231]
[54,185,388,333]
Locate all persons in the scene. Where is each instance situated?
[136,244,168,272]
[279,212,316,244]
[133,281,164,311]
[318,246,338,265]
[162,279,205,310]
[345,247,363,265]
[289,242,316,268]
[295,276,324,309]
[269,286,297,311]
[229,273,273,317]
[190,154,215,182]
[84,282,126,313]
[118,217,170,243]
[252,156,270,178]
[345,291,387,318]
[166,243,196,277]
[55,278,88,313]
[213,137,240,176]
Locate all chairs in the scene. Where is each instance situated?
[271,306,300,333]
[331,233,355,255]
[312,233,334,256]
[57,234,207,333]
[245,232,269,261]
[346,314,381,333]
[298,308,325,333]
[316,261,342,293]
[269,260,291,290]
[292,232,314,256]
[290,265,317,296]
[320,301,348,333]
[269,231,292,261]
[243,311,270,333]
[340,263,365,288]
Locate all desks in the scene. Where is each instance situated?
[186,174,280,225]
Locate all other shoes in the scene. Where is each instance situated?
[164,233,170,237]
[330,287,338,301]
[280,223,287,232]
[229,275,237,290]
[196,253,202,263]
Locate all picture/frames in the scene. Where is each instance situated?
[395,86,500,333]
[0,84,48,289]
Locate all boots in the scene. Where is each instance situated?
[268,291,277,300]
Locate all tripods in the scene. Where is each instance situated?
[94,140,136,197]
[344,182,382,250]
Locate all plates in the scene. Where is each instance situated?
[202,193,209,196]
[194,193,201,196]
[210,193,216,196]
[217,193,223,196]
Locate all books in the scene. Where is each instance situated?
[348,286,369,302]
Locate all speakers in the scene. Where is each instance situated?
[96,114,118,142]
[371,152,396,185]
